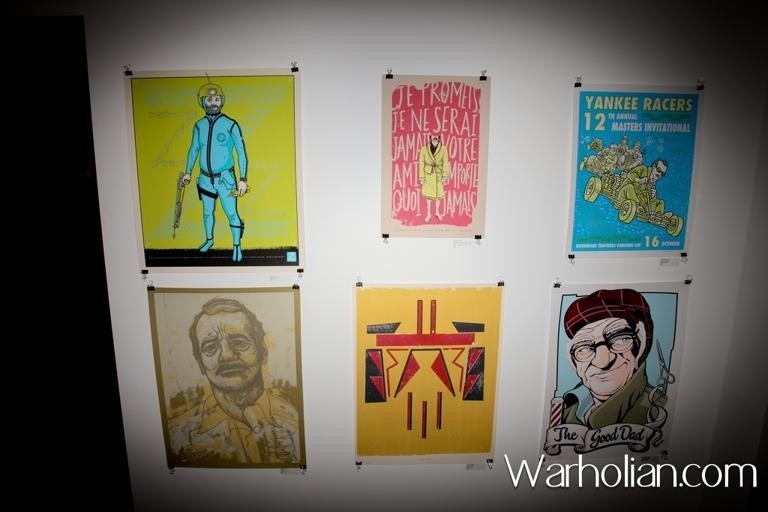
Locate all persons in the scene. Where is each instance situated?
[182,82,247,263]
[594,131,668,205]
[560,289,662,449]
[162,298,298,463]
[418,129,448,223]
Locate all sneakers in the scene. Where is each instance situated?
[199,239,214,252]
[232,244,243,263]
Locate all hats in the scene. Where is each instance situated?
[564,289,654,340]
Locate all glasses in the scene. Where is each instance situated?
[656,165,666,178]
[568,327,642,363]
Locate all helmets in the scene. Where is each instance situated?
[197,83,225,107]
